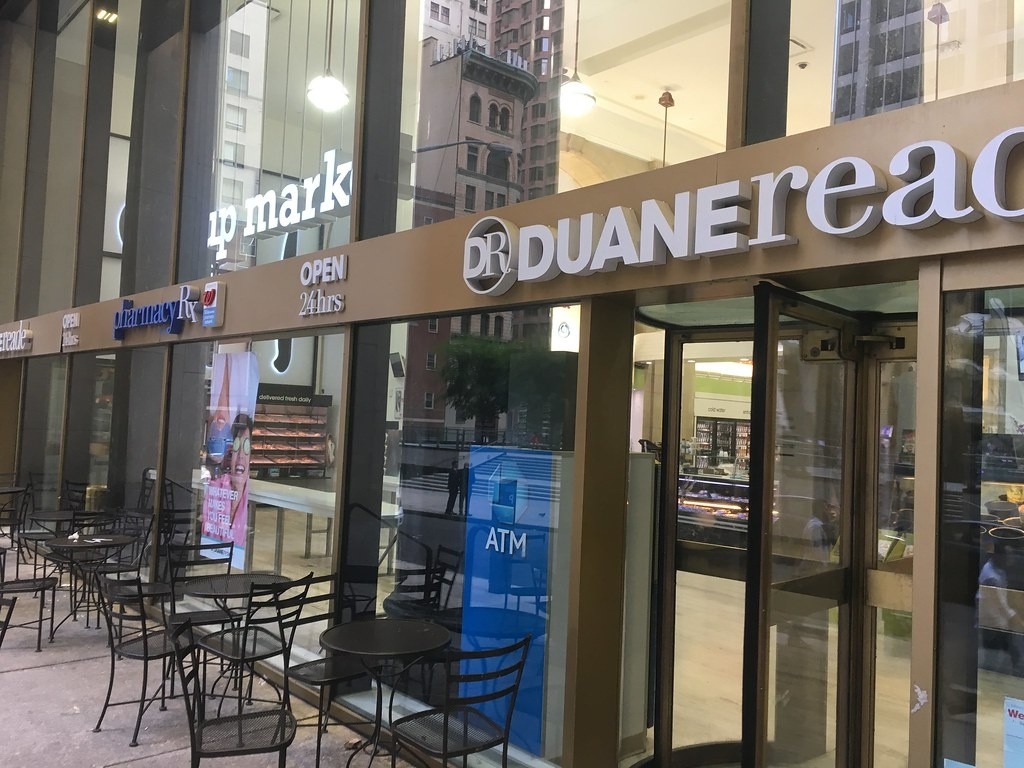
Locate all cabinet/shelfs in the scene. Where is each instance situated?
[247,409,328,476]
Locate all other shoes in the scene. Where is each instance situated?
[459,513,463,516]
[465,512,472,516]
[445,511,457,515]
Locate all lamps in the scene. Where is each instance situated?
[560,0,595,115]
[307,0,350,113]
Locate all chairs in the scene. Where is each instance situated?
[0,469,532,768]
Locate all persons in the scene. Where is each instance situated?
[786,497,832,647]
[972,540,1024,679]
[445,460,476,516]
[202,352,259,549]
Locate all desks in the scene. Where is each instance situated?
[46,534,138,643]
[318,617,453,768]
[438,605,546,759]
[0,487,26,516]
[28,511,103,598]
[174,574,290,747]
[203,475,400,607]
